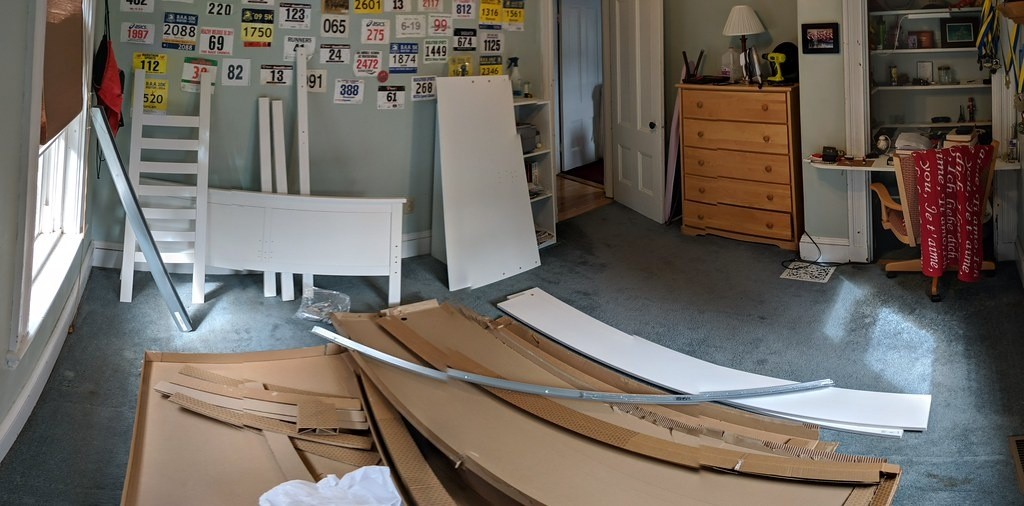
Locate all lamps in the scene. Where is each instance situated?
[722,4,766,84]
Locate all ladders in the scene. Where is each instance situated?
[120,68,212,303]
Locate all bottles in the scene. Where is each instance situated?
[938,66,951,83]
[722,47,741,83]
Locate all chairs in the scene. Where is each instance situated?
[868,139,1000,303]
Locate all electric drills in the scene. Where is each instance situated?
[761,52,787,86]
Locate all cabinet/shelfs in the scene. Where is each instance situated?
[675,77,805,251]
[429,95,559,266]
[865,3,996,155]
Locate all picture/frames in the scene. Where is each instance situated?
[939,16,981,48]
[801,22,839,54]
